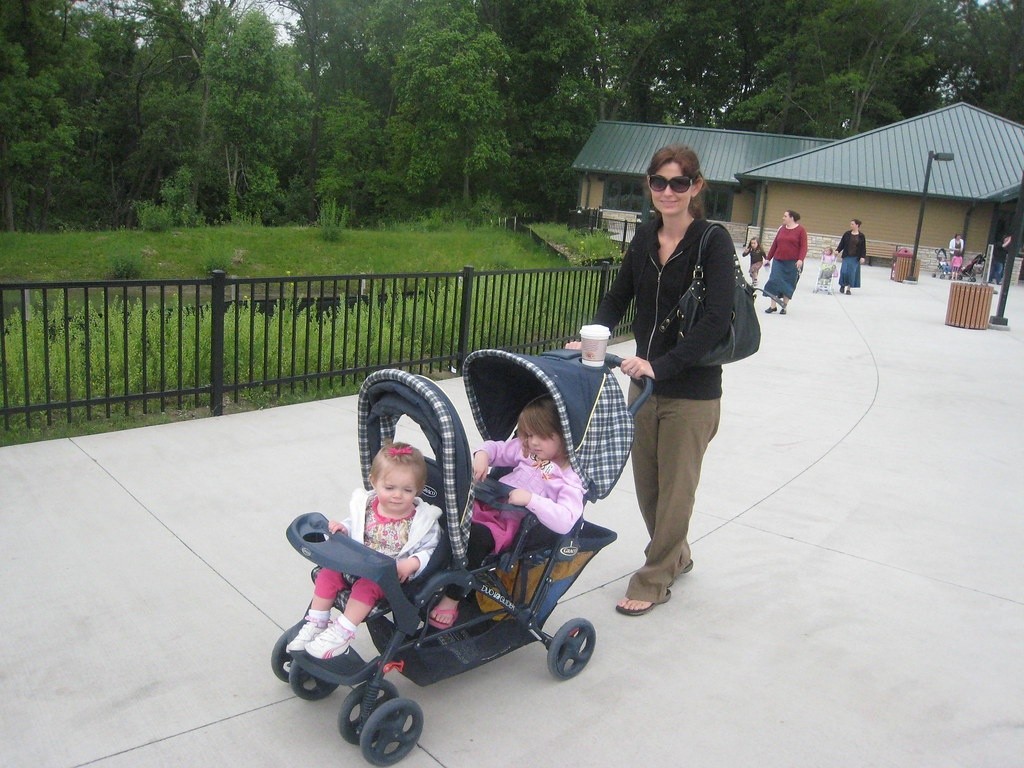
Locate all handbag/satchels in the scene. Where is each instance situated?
[658,223,761,367]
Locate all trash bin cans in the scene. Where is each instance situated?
[945,282,994,330]
[895,257,920,283]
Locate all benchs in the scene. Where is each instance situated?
[864,241,901,266]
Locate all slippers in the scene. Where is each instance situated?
[677,559,695,576]
[615,586,673,617]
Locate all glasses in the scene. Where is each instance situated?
[646,172,699,193]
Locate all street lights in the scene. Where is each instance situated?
[905,150,954,281]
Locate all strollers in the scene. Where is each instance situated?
[813,252,840,295]
[934,248,952,279]
[270,323,657,767]
[956,251,988,283]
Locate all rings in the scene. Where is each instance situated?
[629,370,634,374]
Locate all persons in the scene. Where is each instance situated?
[761,209,808,314]
[286,443,444,662]
[950,249,963,280]
[428,395,583,630]
[949,233,964,259]
[834,219,866,295]
[820,246,838,279]
[987,232,1012,284]
[565,144,735,616]
[742,237,766,285]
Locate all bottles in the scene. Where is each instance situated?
[765,263,769,273]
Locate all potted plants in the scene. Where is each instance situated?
[1004,252,1024,286]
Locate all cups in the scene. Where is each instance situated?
[580,324,611,367]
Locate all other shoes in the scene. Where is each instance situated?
[305,624,351,660]
[286,620,332,653]
[998,281,1002,285]
[753,278,759,288]
[846,290,851,296]
[780,309,787,314]
[840,287,844,293]
[990,281,995,284]
[766,306,777,313]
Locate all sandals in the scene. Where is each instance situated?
[427,603,462,631]
